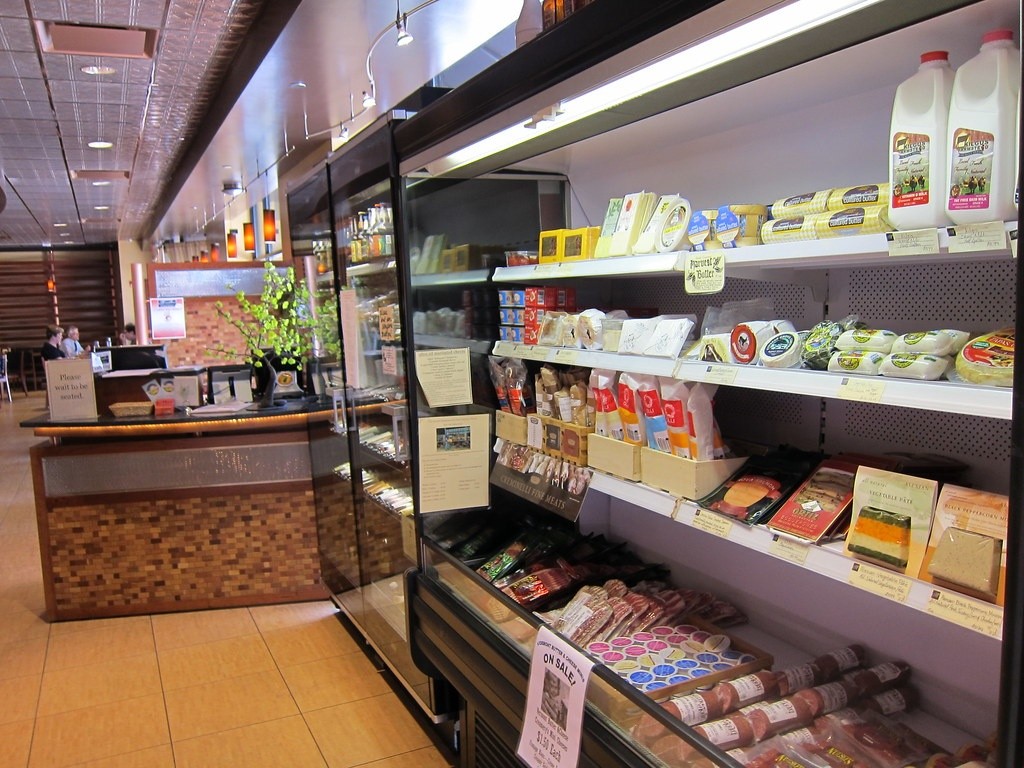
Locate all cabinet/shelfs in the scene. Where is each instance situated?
[493,218,1020,642]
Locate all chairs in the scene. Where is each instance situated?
[0,348,50,409]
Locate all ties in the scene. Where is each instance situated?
[74,343,77,352]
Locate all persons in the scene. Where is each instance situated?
[41,325,64,360]
[541,671,567,723]
[120,324,136,345]
[59,326,91,357]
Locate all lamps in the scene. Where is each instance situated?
[227,234,237,258]
[192,243,221,262]
[361,91,375,107]
[243,223,255,252]
[395,19,414,47]
[263,209,275,243]
[339,127,349,139]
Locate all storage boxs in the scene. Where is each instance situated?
[539,224,601,262]
[496,409,746,500]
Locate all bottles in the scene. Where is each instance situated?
[945,30,1020,226]
[889,50,956,229]
[339,201,392,264]
